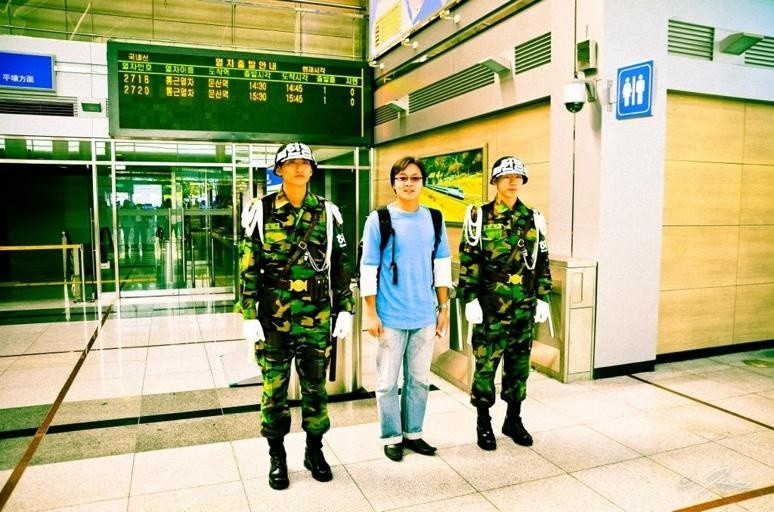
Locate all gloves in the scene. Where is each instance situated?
[535,299,550,323]
[244,319,266,344]
[332,312,352,340]
[465,298,484,326]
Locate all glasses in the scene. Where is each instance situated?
[395,175,423,183]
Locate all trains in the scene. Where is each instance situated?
[427,183,464,200]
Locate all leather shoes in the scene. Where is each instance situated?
[404,436,437,455]
[383,442,402,461]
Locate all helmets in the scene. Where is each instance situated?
[490,157,528,185]
[273,142,317,177]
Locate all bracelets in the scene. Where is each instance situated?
[437,304,449,309]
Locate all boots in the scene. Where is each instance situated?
[304,432,333,482]
[503,402,533,446]
[477,408,496,450]
[268,438,288,489]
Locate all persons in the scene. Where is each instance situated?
[236,142,357,490]
[355,157,456,462]
[456,154,556,449]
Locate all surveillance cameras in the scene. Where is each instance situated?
[563,83,586,112]
